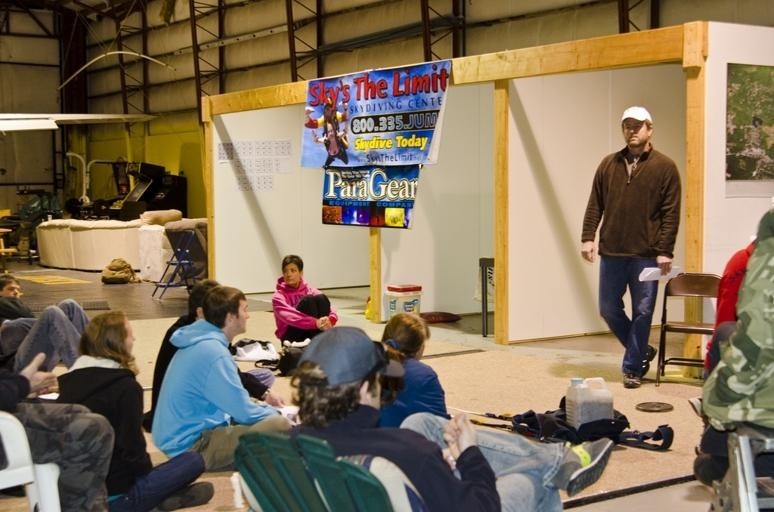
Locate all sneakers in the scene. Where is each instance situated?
[552,436,616,500]
[624,374,641,389]
[160,480,215,512]
[642,345,658,377]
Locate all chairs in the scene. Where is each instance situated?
[655,273,724,387]
[0,411,60,512]
[231,426,430,512]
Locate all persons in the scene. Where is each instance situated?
[694,206,773,485]
[304,98,349,146]
[580,107,682,390]
[311,121,349,166]
[686,237,754,419]
[1,253,615,510]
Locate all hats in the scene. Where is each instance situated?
[296,325,390,389]
[619,106,653,126]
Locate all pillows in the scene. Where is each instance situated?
[420,310,462,323]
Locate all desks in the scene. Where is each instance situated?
[473,257,495,337]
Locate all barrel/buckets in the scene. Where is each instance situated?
[564,375,616,432]
